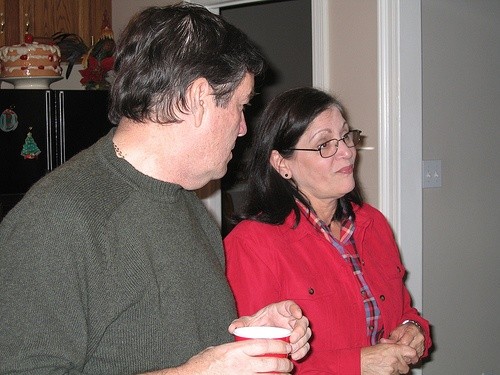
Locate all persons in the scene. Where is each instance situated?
[223,87,431,375]
[1,3,270,375]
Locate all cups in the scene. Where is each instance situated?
[233,326,292,375]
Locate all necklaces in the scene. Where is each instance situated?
[113,143,125,158]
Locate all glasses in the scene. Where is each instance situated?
[286,129,361,158]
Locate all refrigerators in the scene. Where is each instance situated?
[0,85,117,230]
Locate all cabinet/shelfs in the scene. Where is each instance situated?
[0,0,115,65]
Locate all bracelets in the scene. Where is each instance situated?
[402,320,422,330]
